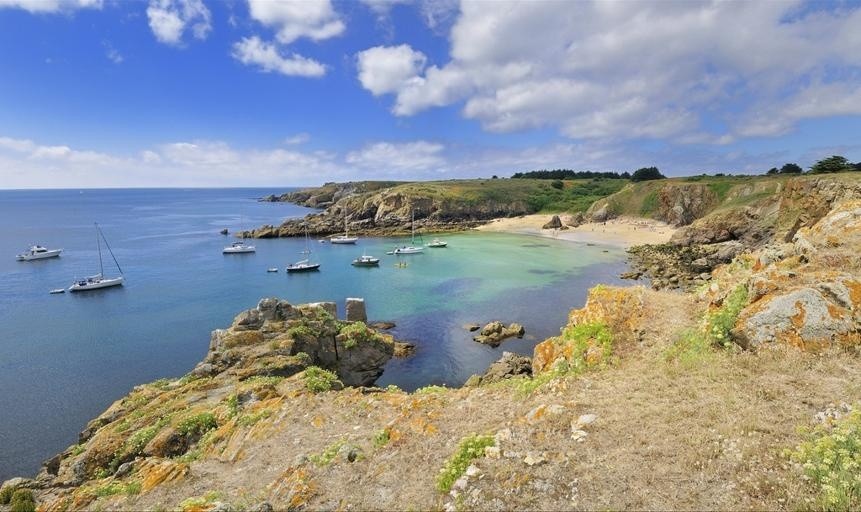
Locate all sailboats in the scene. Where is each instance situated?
[68,220,131,292]
[331,204,359,244]
[286,224,321,270]
[221,197,258,253]
[394,205,426,255]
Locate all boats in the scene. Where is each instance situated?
[423,238,448,248]
[16,243,64,262]
[386,251,394,256]
[48,287,65,294]
[350,247,382,267]
[317,238,327,245]
[267,266,279,272]
[393,263,409,267]
